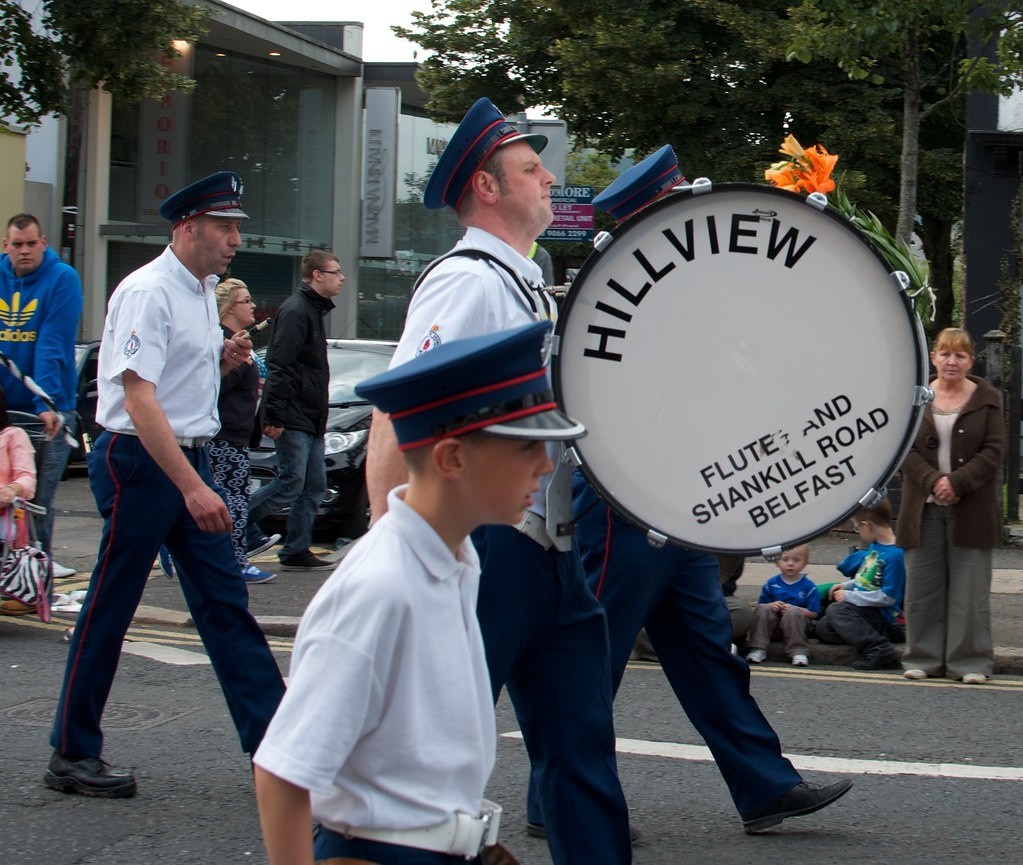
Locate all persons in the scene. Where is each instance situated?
[634,557,753,662]
[0,214,82,616]
[0,380,36,558]
[42,172,287,798]
[365,97,633,865]
[248,250,345,571]
[525,144,854,842]
[896,327,1006,684]
[156,277,277,584]
[252,320,589,865]
[745,543,821,666]
[814,490,906,671]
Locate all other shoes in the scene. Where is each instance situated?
[245,534,281,559]
[963,672,984,683]
[281,550,335,571]
[52,561,77,577]
[0,597,28,614]
[905,667,927,679]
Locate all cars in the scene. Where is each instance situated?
[66,340,104,476]
[243,339,399,546]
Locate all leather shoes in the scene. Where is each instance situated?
[523,819,642,842]
[42,751,135,796]
[743,781,849,830]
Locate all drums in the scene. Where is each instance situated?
[549,181,933,559]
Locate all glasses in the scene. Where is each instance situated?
[236,298,254,304]
[314,269,343,277]
[852,521,870,533]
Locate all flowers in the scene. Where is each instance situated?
[763,133,939,318]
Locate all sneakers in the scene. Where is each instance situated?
[158,544,174,580]
[747,650,765,662]
[240,564,276,585]
[793,652,808,665]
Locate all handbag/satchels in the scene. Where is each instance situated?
[0,497,51,622]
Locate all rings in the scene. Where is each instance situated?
[5,497,9,500]
[232,353,237,357]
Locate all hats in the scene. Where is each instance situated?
[422,97,547,212]
[161,171,250,227]
[353,323,586,451]
[591,144,690,222]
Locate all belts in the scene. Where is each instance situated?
[106,427,208,448]
[510,509,551,549]
[320,796,502,860]
